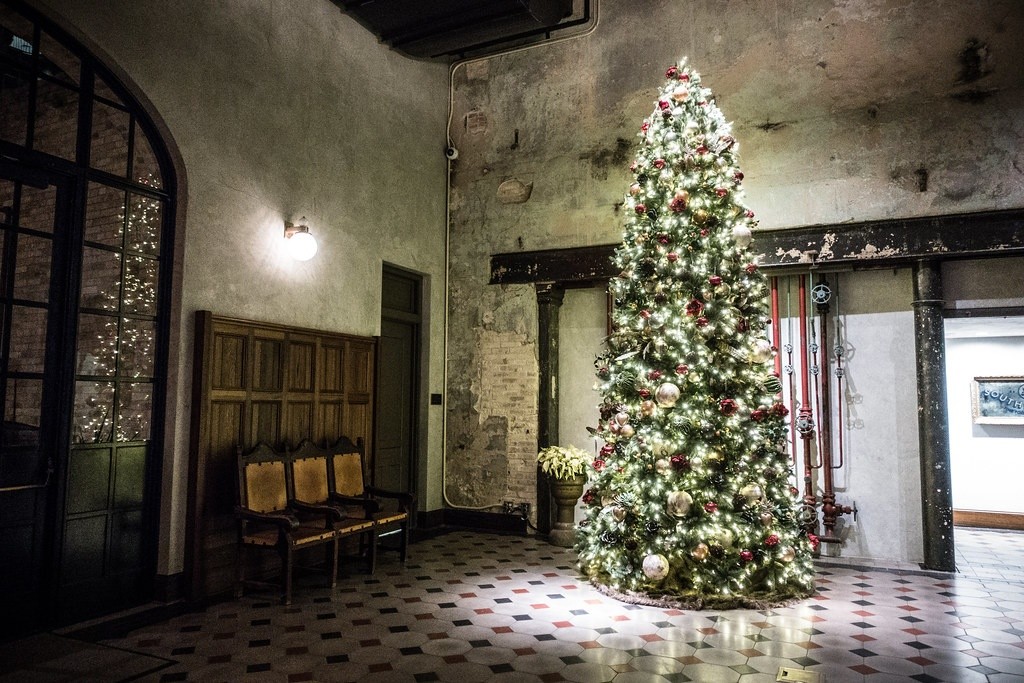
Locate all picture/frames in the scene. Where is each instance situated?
[970,375,1024,425]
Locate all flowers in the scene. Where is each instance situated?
[531,443,596,483]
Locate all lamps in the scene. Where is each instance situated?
[283,219,317,262]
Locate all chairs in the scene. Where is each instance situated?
[234,434,417,607]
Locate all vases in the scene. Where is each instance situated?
[547,473,595,548]
